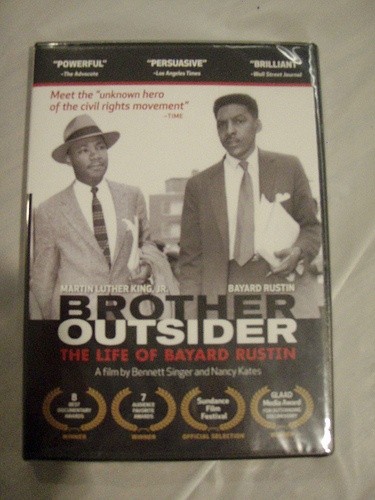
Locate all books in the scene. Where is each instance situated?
[19,39,336,464]
[121,214,141,276]
[252,199,301,282]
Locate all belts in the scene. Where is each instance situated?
[247,251,284,265]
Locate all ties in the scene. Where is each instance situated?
[234,160,254,264]
[91,186,110,270]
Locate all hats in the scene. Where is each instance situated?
[50,115,119,165]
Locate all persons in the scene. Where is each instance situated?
[25,114,166,320]
[177,94,322,323]
